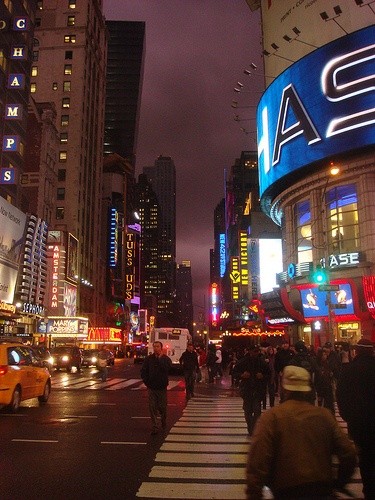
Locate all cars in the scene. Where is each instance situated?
[0,342,52,412]
[134,350,147,364]
[81,349,116,369]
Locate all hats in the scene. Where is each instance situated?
[282,365,312,391]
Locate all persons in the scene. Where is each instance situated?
[334,290,347,304]
[135,348,141,356]
[38,335,44,345]
[229,346,275,411]
[306,288,317,306]
[274,340,357,419]
[335,339,375,500]
[96,345,108,382]
[196,346,228,385]
[179,343,198,401]
[140,341,172,432]
[245,365,356,500]
[67,289,75,317]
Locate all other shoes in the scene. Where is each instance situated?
[186,394,192,400]
[151,430,158,435]
[161,428,167,435]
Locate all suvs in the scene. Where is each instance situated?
[47,345,82,372]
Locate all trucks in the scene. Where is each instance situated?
[148,327,191,364]
